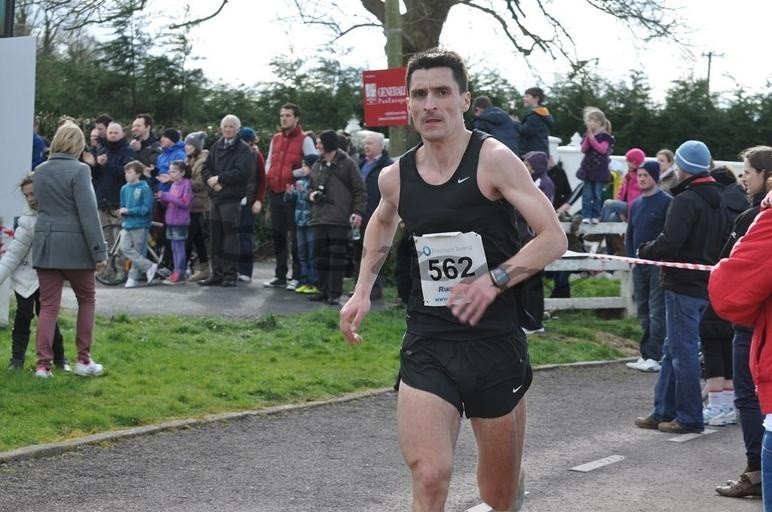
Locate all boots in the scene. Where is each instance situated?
[188,261,211,281]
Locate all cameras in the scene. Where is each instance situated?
[314,192,327,204]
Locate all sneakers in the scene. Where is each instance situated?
[156,267,171,277]
[238,275,251,282]
[582,216,599,224]
[125,275,139,288]
[7,358,26,371]
[163,272,186,286]
[286,278,299,290]
[724,406,738,424]
[625,357,645,370]
[54,360,73,373]
[521,323,545,334]
[702,406,727,426]
[637,358,661,373]
[74,357,104,377]
[35,368,55,379]
[146,263,158,284]
[263,277,286,288]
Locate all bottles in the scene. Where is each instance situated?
[351,214,361,241]
[147,233,157,248]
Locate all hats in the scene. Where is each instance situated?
[302,154,319,166]
[239,127,256,142]
[637,161,661,183]
[184,130,208,151]
[162,128,180,143]
[674,140,711,173]
[656,148,675,163]
[626,147,645,165]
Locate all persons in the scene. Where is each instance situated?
[33,118,50,168]
[0,170,71,372]
[57,88,771,374]
[713,141,771,498]
[338,48,568,511]
[32,117,108,376]
[634,140,732,433]
[706,191,771,511]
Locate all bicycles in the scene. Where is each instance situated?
[92,195,216,288]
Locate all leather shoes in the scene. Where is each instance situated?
[295,273,383,305]
[715,471,762,500]
[198,275,237,286]
[658,418,704,434]
[634,411,673,429]
